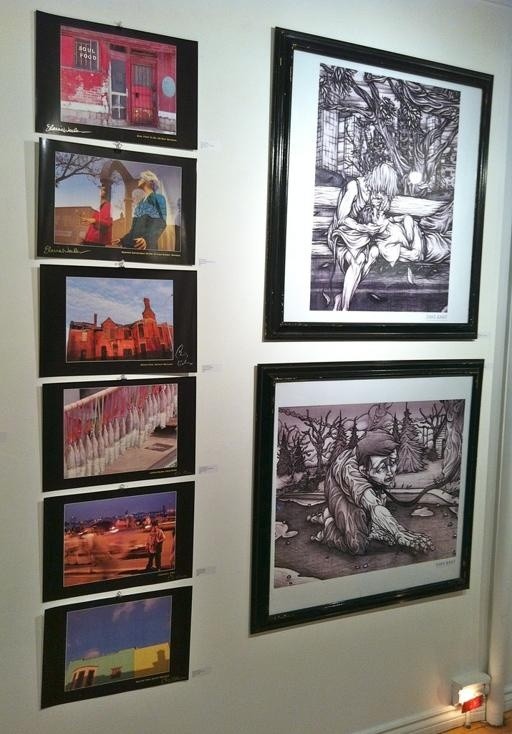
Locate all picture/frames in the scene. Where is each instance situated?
[40,376,197,492]
[41,586,194,710]
[37,136,198,265]
[247,356,484,637]
[33,9,198,151]
[40,481,196,602]
[38,262,199,375]
[262,25,495,342]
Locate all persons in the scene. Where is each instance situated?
[80,170,169,251]
[305,428,436,554]
[326,162,454,312]
[145,519,166,569]
